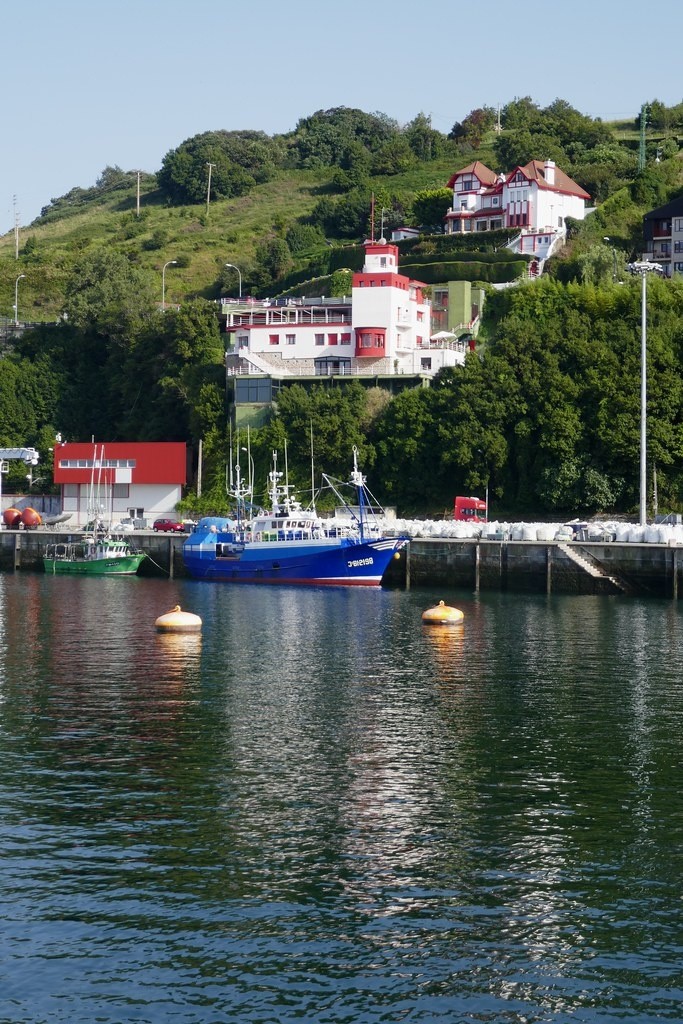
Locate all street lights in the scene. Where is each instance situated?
[14,274,25,322]
[161,260,177,308]
[225,263,242,299]
[626,256,662,526]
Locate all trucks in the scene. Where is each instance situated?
[453,496,488,523]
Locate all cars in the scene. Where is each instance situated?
[153,518,185,533]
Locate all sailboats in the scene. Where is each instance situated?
[42,444,147,576]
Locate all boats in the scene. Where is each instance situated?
[183,416,412,588]
